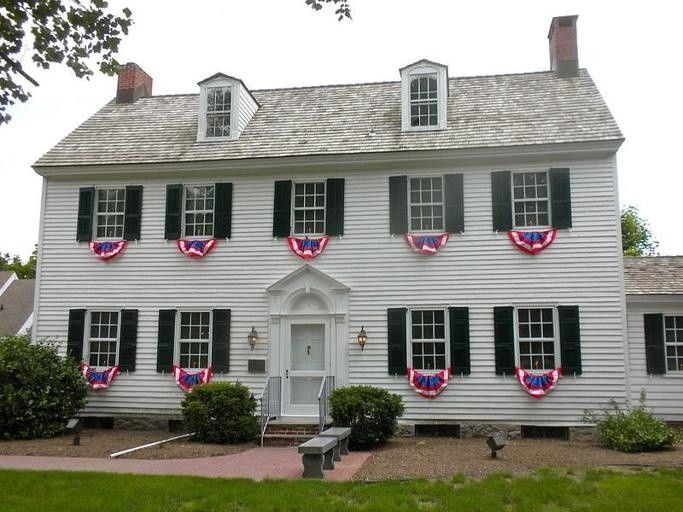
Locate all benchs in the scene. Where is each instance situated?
[319,427,351,461]
[298,437,337,479]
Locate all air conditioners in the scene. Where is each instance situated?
[248,358,267,373]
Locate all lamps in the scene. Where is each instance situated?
[357,326,367,351]
[248,326,258,351]
[486,435,504,457]
[66,418,80,445]
